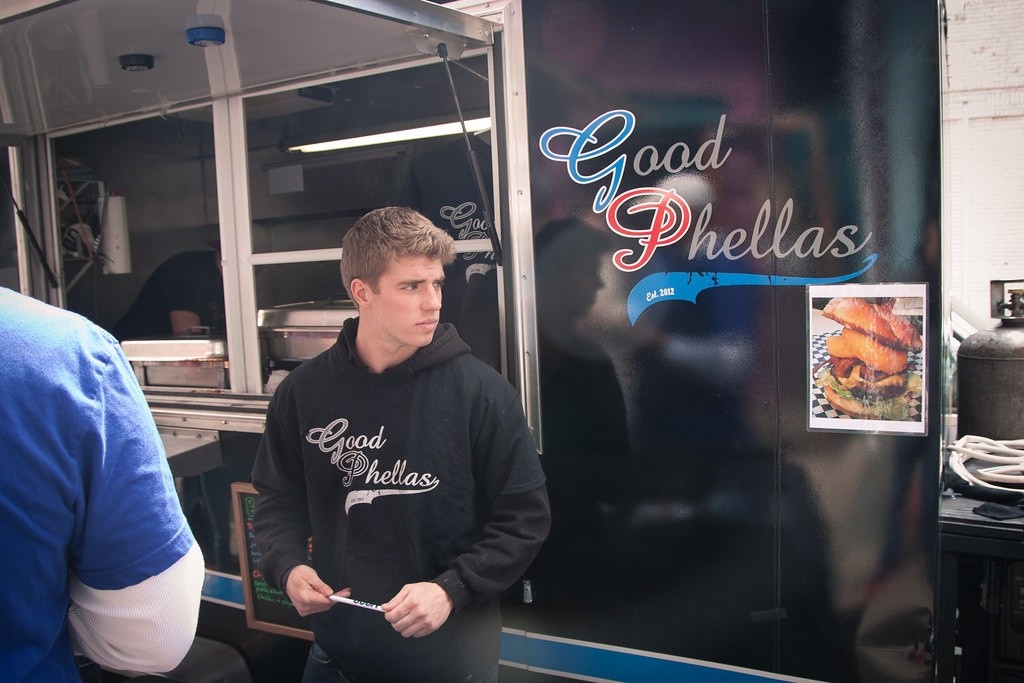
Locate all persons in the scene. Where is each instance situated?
[0,285,205,683]
[254,209,552,683]
[118,226,272,571]
[537,176,937,683]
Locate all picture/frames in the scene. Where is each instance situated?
[805,282,929,437]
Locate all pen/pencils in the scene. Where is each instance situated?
[328,595,385,612]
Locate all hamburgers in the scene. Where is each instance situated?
[811,298,923,423]
